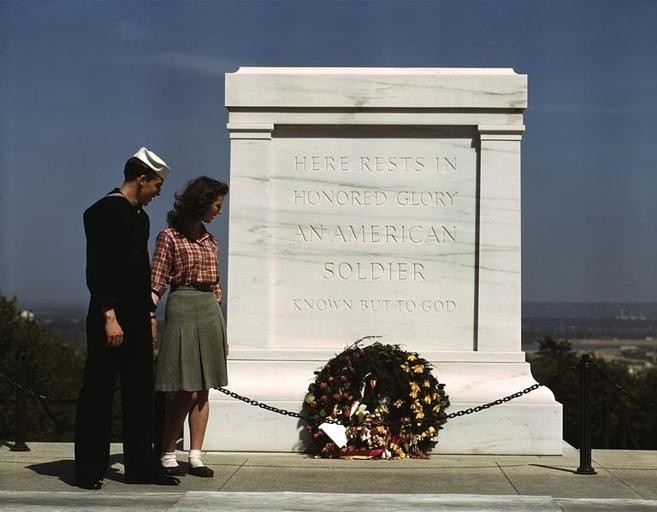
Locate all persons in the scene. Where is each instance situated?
[148,177,229,478]
[72,144,181,488]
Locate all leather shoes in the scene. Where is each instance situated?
[161,461,184,476]
[125,471,179,484]
[188,461,214,477]
[85,470,102,489]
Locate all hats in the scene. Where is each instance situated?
[133,147,171,180]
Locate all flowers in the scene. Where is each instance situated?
[300,334,451,460]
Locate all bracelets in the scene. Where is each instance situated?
[149,315,156,320]
